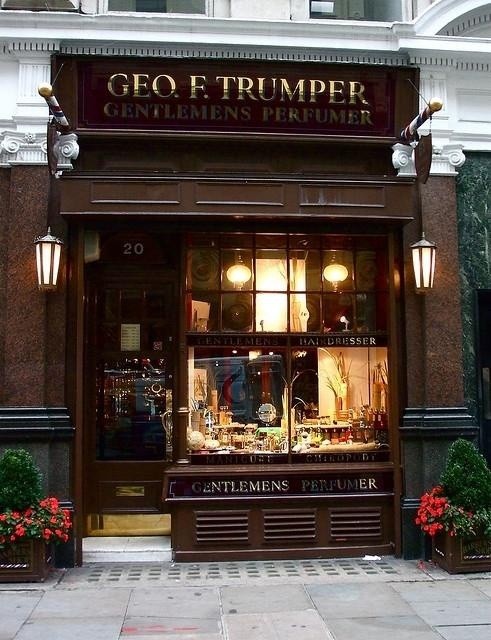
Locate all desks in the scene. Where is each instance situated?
[295,423,352,441]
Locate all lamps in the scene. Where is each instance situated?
[410,232,438,294]
[34,226,65,292]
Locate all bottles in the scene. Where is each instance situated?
[197,398,388,450]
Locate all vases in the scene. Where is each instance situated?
[0,537,55,582]
[431,529,491,574]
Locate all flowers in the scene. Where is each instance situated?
[0,449,73,547]
[415,438,491,536]
